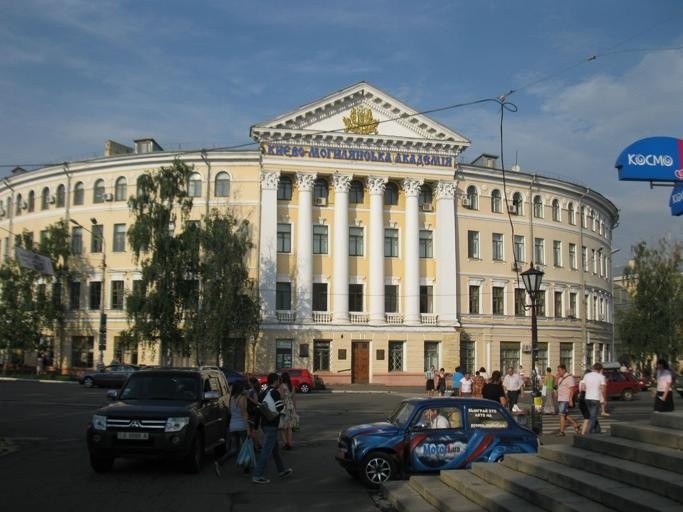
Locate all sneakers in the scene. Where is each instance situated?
[555,424,582,438]
[251,475,272,485]
[213,460,225,479]
[277,468,294,481]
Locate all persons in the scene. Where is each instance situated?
[111,355,118,370]
[655,358,673,412]
[213,372,299,484]
[36,352,44,376]
[424,363,651,436]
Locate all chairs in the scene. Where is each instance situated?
[448,412,460,428]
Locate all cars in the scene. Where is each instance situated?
[80,360,141,388]
[553,362,641,401]
[634,375,651,391]
[220,366,249,392]
[674,375,682,396]
[335,396,543,488]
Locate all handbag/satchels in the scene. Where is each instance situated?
[236,438,259,469]
[255,387,284,424]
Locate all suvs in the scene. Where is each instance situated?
[86,364,232,474]
[256,368,315,393]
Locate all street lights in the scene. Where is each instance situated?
[579,245,621,372]
[520,261,547,432]
[70,216,107,371]
